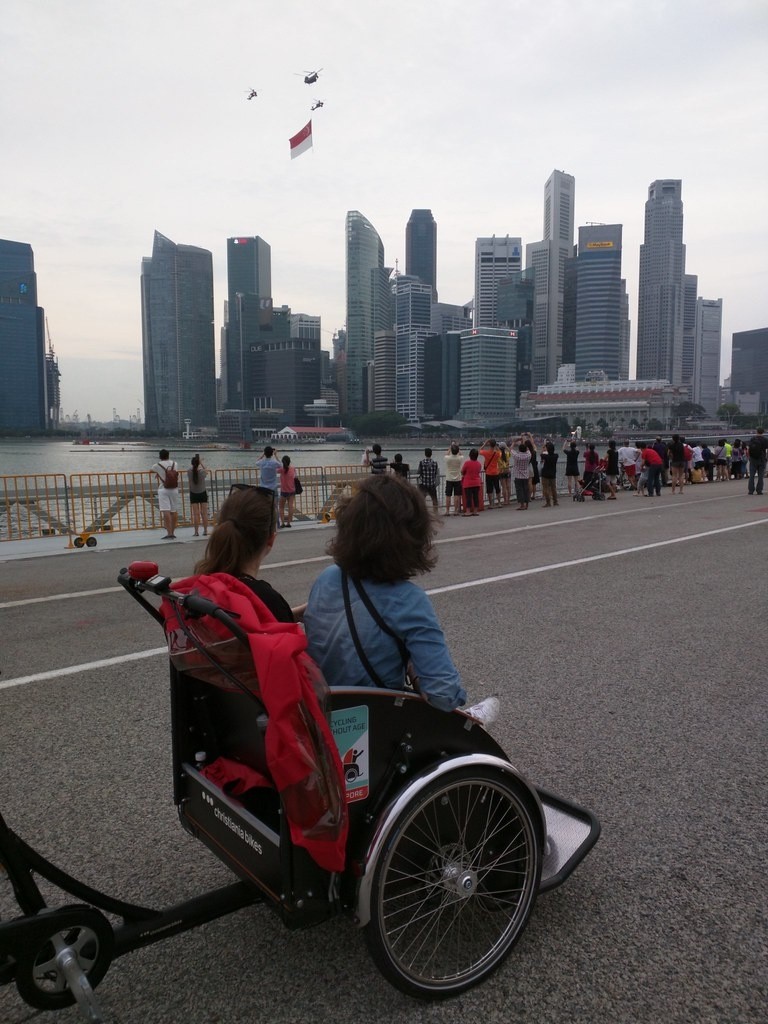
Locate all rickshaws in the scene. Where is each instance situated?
[0,566,602,1024]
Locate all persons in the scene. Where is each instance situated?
[418,448,439,514]
[618,440,640,489]
[444,444,464,516]
[278,455,304,527]
[524,440,533,501]
[603,439,618,500]
[185,485,498,731]
[306,472,469,715]
[583,443,599,488]
[520,432,540,499]
[748,426,768,494]
[510,438,532,510]
[633,460,647,497]
[257,447,280,530]
[390,455,410,480]
[540,442,559,508]
[187,453,209,537]
[669,435,684,494]
[638,441,662,496]
[367,445,388,474]
[634,434,768,486]
[563,438,580,497]
[495,441,511,505]
[463,450,482,516]
[478,438,502,510]
[151,449,181,540]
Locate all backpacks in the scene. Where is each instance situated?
[684,444,691,461]
[157,461,178,488]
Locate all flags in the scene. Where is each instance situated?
[290,119,313,160]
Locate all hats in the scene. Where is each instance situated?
[499,442,507,450]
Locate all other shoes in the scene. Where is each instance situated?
[285,524,291,527]
[202,531,208,536]
[161,535,171,540]
[193,532,199,536]
[280,524,285,528]
[172,535,177,540]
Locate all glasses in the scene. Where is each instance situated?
[229,483,276,536]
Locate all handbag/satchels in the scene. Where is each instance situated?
[360,449,372,467]
[294,469,303,494]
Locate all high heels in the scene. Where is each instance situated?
[464,697,499,733]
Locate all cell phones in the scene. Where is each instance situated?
[272,447,275,451]
[524,434,528,436]
[369,449,373,453]
[196,454,199,459]
